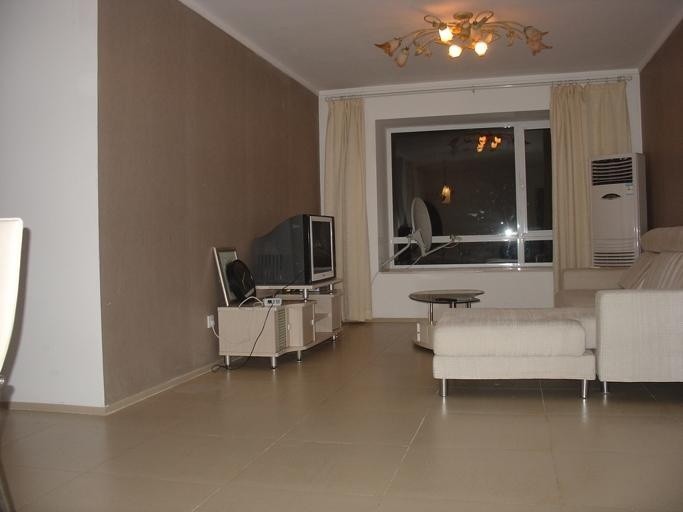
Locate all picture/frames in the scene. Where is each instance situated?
[212,246,240,306]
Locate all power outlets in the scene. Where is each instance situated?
[207,313,215,328]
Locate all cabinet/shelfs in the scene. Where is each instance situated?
[218,278,345,368]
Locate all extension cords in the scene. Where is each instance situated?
[263,298,282,307]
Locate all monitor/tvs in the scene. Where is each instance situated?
[252,213,336,286]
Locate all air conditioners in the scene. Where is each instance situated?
[588,153,646,269]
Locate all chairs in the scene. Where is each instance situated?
[0,217,23,512]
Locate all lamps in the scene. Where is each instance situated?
[373,10,554,68]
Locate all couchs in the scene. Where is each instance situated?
[432,226,683,399]
